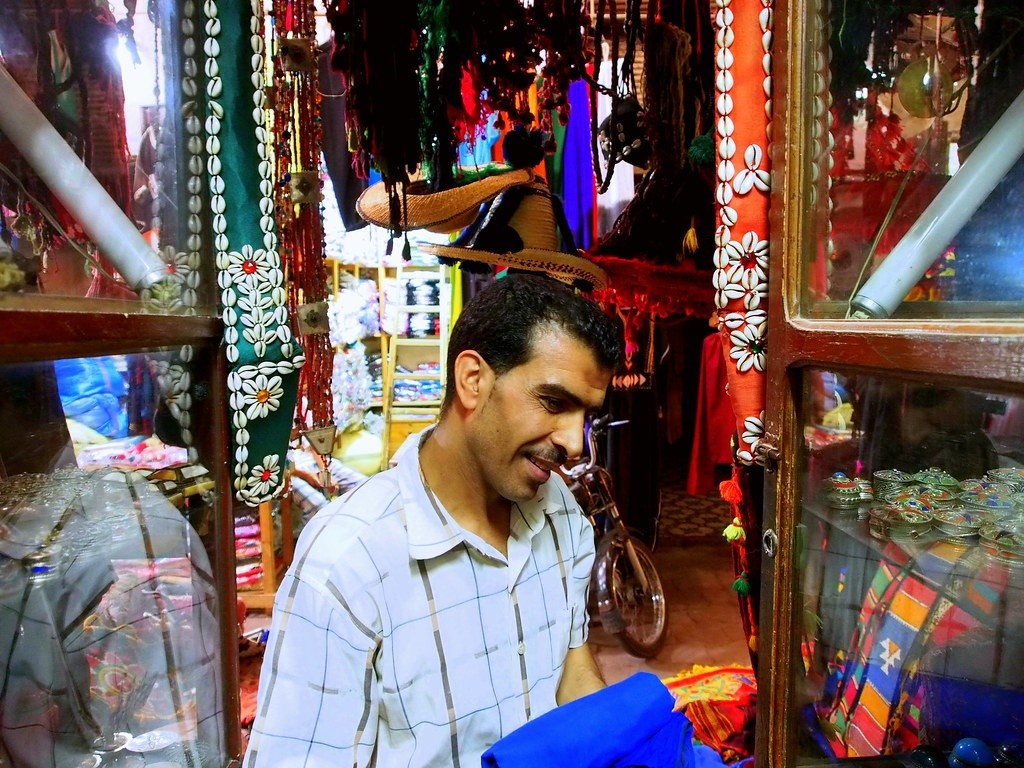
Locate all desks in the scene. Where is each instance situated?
[234,462,296,612]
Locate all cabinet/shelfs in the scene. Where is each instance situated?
[323,259,458,476]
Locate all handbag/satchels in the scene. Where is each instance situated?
[599,95,654,171]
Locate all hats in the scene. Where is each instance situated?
[414,171,603,288]
[354,165,535,234]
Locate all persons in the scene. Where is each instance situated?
[0,360,228,768]
[241,271,626,768]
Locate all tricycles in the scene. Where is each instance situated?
[311,264,670,656]
